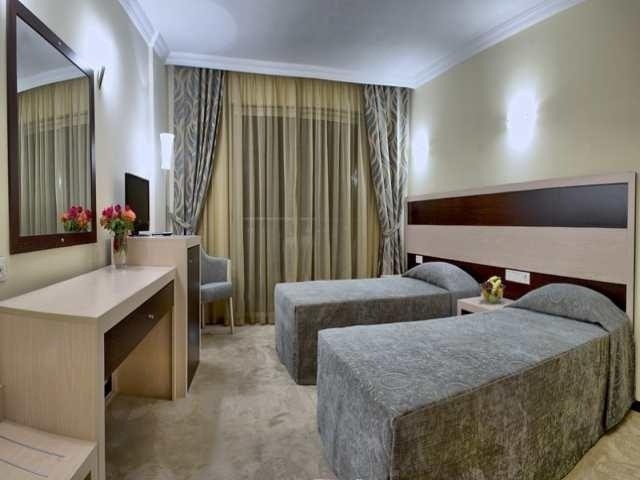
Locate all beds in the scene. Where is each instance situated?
[271,260,483,387]
[316,280,640,480]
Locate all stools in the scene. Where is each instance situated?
[0,413,101,480]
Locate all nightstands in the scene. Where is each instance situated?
[456,293,515,316]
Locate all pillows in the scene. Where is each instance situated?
[516,280,619,327]
[402,261,480,294]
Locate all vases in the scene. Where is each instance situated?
[482,287,505,304]
[112,234,129,270]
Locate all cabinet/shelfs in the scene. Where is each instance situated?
[114,233,201,401]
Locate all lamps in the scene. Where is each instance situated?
[159,133,175,170]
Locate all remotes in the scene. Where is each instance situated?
[152,233,172,236]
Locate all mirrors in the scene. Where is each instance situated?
[5,0,97,257]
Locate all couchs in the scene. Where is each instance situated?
[198,245,236,337]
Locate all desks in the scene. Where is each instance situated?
[0,263,178,480]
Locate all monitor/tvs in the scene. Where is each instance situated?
[124,173,150,236]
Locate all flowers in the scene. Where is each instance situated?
[100,202,136,256]
[60,205,93,234]
[481,275,506,295]
